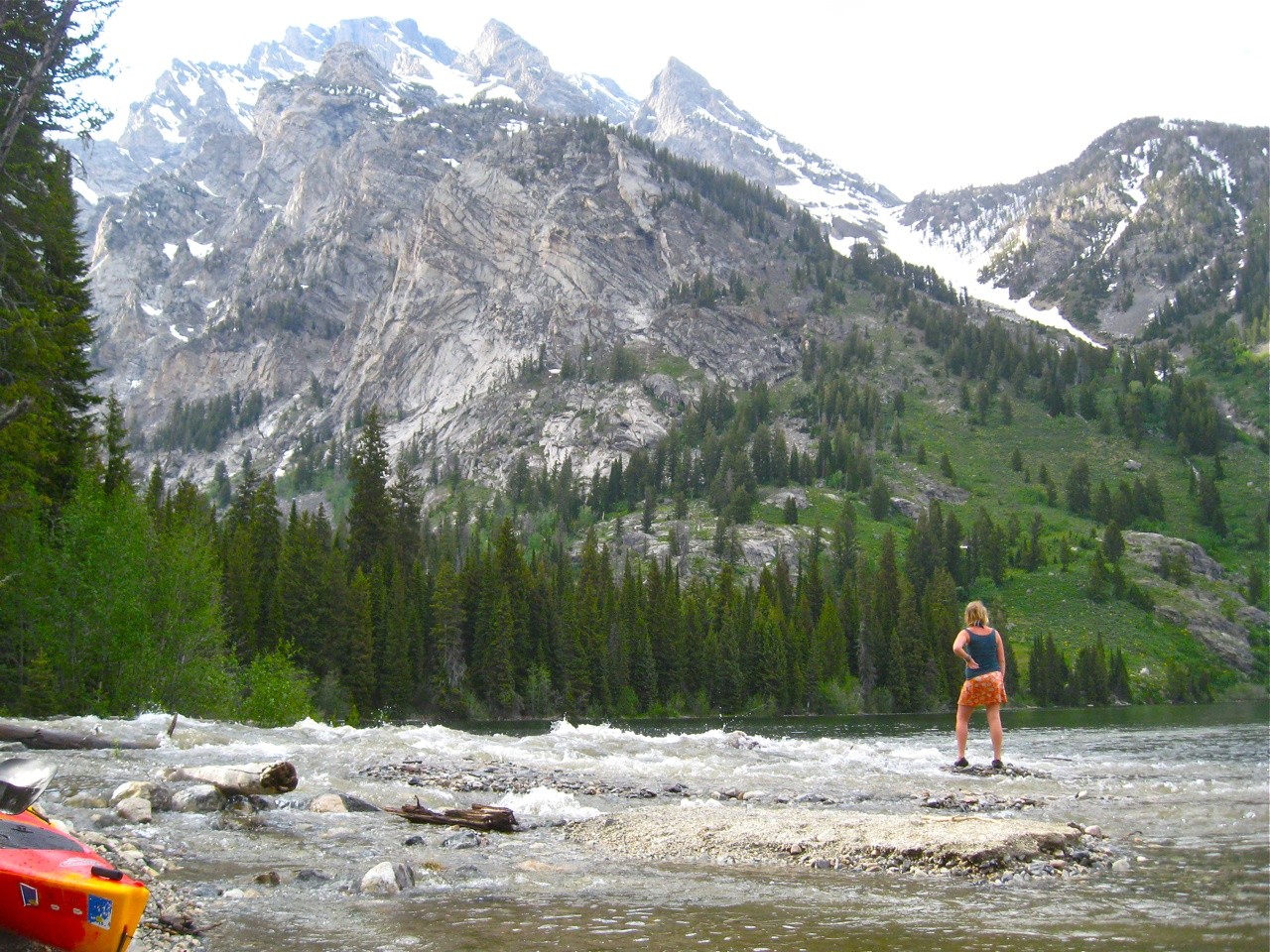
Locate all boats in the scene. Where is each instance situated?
[1,804,147,952]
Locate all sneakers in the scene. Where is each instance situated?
[991,760,1003,768]
[953,757,969,767]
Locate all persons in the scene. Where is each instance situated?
[954,600,1006,767]
